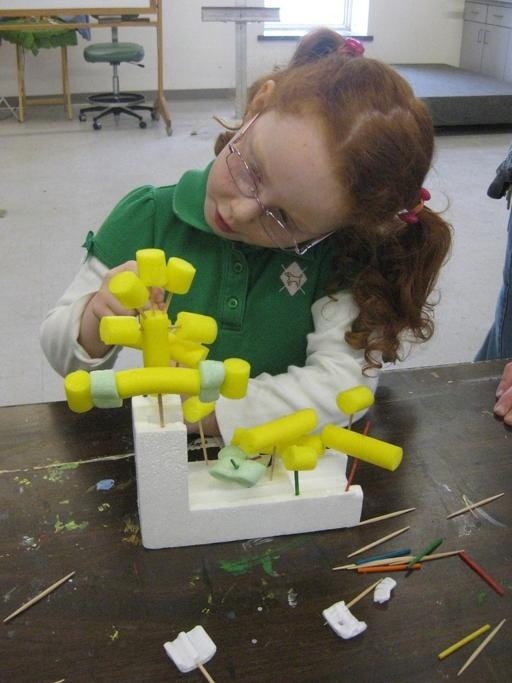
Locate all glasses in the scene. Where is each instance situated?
[224,109,336,257]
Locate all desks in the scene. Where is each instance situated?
[1,0,176,135]
[1,358,512,683]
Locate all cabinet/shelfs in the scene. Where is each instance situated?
[459,1,512,82]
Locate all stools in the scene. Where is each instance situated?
[78,41,160,131]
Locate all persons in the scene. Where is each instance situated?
[36,29,452,446]
[468,138,512,427]
